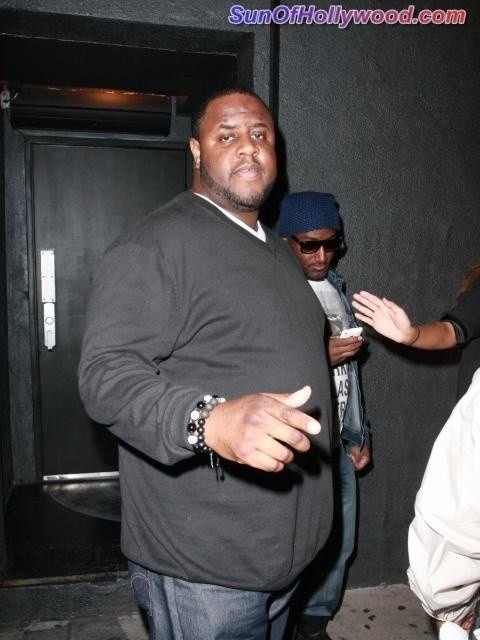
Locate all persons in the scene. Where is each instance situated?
[408,367,480,640]
[351,263,480,405]
[272,191,371,640]
[78,86,335,640]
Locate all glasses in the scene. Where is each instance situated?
[292,234,344,254]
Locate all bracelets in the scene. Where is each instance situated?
[408,325,420,346]
[187,394,226,484]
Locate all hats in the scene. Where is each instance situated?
[276,191,341,237]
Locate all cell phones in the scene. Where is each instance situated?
[341,327,363,339]
